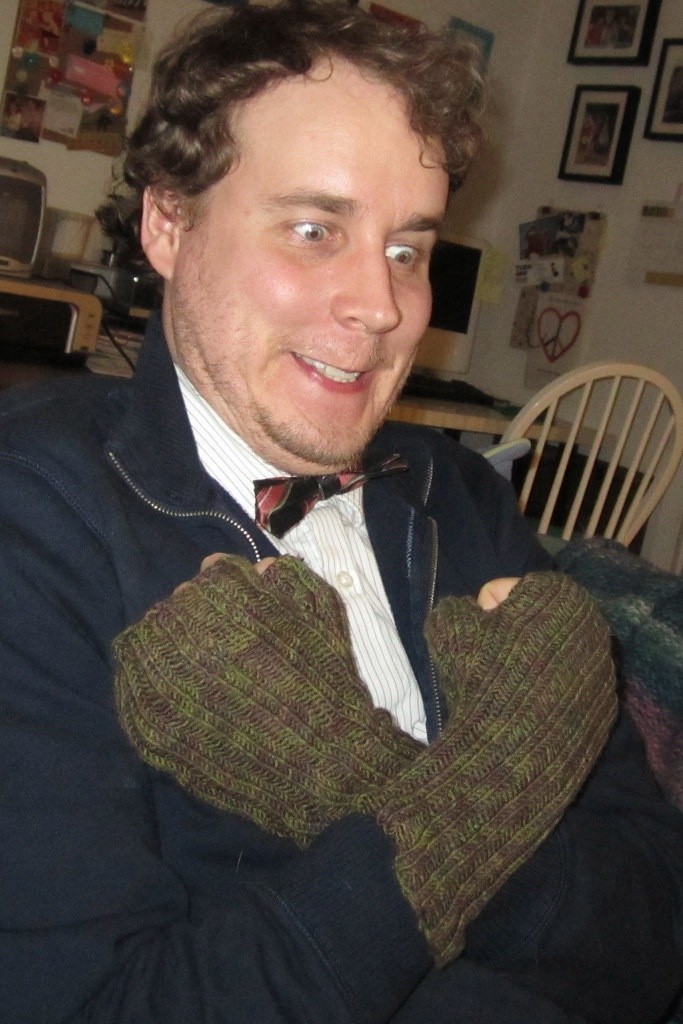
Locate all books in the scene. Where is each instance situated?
[89,327,143,378]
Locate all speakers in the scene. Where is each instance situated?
[0,156,47,279]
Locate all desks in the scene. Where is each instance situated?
[0,276,624,448]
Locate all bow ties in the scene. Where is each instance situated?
[252,450,410,540]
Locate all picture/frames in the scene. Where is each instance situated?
[567,0,663,67]
[643,37,683,142]
[558,83,642,186]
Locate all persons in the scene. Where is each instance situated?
[0,0,682,1023]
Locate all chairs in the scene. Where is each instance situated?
[499,359,683,548]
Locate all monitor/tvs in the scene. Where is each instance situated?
[411,230,492,375]
